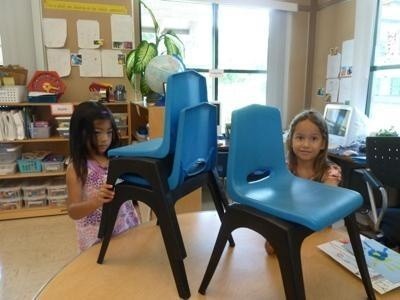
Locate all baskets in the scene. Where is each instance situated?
[0,86,26,103]
[17,151,51,173]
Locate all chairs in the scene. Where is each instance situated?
[362,137,400,248]
[97,68,237,299]
[196,100,376,300]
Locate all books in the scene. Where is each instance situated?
[0,107,128,212]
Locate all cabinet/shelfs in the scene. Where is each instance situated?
[0,100,133,221]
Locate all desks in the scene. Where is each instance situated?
[35,210,400,300]
[327,152,369,190]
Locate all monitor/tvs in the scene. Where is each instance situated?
[323,104,371,149]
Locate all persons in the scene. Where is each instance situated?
[281,109,343,186]
[66,100,142,254]
